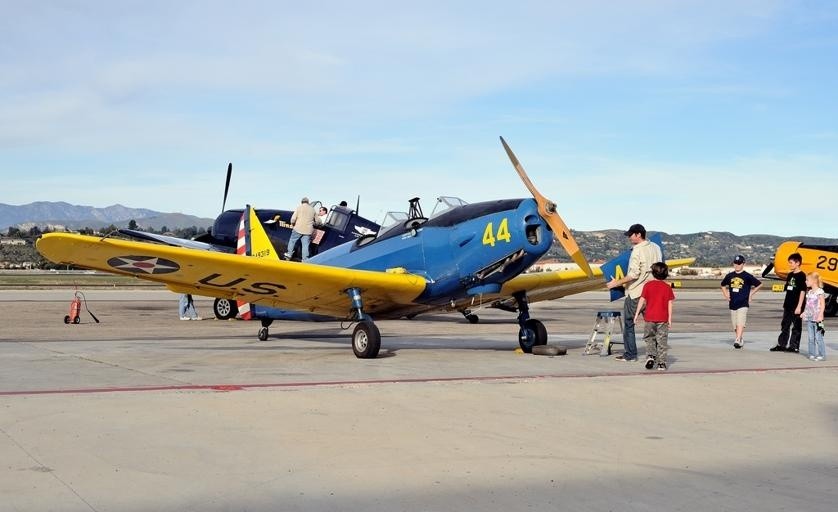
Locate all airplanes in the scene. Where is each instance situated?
[760,239,838,358]
[34,133,698,359]
[119,160,483,323]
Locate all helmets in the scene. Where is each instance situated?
[734,255,745,263]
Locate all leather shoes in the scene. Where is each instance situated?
[770,345,787,351]
[785,347,800,353]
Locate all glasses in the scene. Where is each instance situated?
[735,263,742,265]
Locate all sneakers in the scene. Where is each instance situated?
[191,315,203,320]
[739,339,744,347]
[734,338,741,348]
[284,252,292,258]
[808,353,816,360]
[179,316,190,320]
[616,355,667,371]
[816,356,826,361]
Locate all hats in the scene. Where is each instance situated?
[624,224,645,236]
[302,197,308,201]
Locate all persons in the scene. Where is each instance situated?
[800,272,826,361]
[605,224,662,361]
[178,294,202,321]
[633,262,675,370]
[769,254,807,353]
[279,198,322,262]
[340,201,347,206]
[307,207,329,256]
[720,254,762,348]
